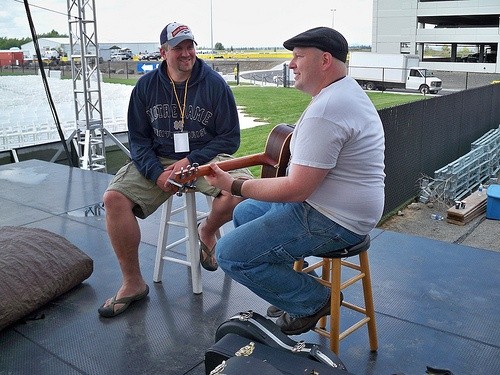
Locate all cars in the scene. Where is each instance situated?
[461,53,485,63]
[110,52,161,61]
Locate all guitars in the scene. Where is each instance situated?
[165,120,296,198]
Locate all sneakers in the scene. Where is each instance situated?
[266,305,284,318]
[276,290,343,336]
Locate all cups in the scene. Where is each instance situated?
[461,201,466,209]
[455,201,461,209]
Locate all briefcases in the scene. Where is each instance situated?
[205,333,352,375]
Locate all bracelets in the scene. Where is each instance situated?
[231,178,249,198]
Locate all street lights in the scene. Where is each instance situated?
[330,9,336,29]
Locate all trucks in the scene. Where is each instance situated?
[349,51,443,94]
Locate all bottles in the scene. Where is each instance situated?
[478,184,483,196]
[430,214,444,221]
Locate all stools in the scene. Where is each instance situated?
[152,187,224,294]
[292,235,378,358]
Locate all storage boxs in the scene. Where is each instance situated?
[485,184,500,220]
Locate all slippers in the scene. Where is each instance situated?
[98,283,149,318]
[197,222,218,271]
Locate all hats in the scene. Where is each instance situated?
[160,21,198,48]
[283,27,348,63]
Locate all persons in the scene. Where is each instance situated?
[204,27,385,336]
[98,23,255,316]
